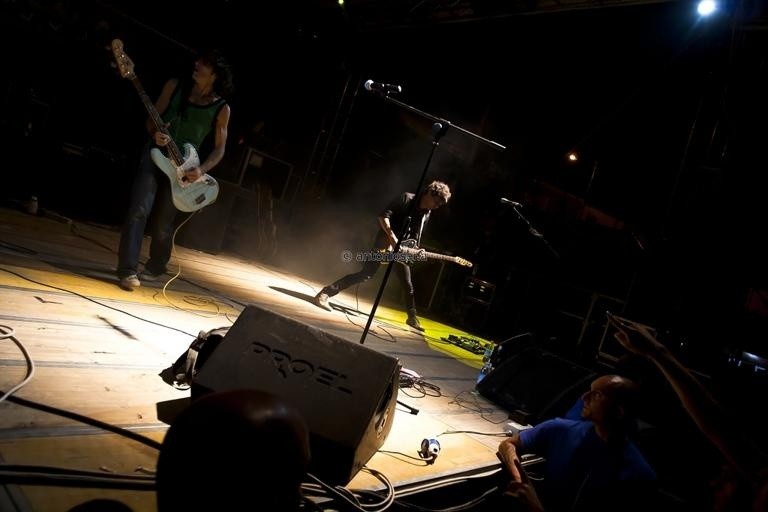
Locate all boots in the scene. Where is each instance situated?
[406,309,425,332]
[314,286,339,311]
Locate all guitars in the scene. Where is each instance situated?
[112,39,220,212]
[375,236,473,268]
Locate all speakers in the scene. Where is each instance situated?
[191,304,403,487]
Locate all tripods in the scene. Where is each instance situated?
[359,126,438,418]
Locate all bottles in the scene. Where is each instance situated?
[476,340,495,384]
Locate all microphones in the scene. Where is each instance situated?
[500,198,523,208]
[364,79,402,94]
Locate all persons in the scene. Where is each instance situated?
[119,44,232,289]
[607,311,762,482]
[499,375,657,512]
[314,179,451,331]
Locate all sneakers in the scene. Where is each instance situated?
[138,266,164,281]
[118,270,140,289]
